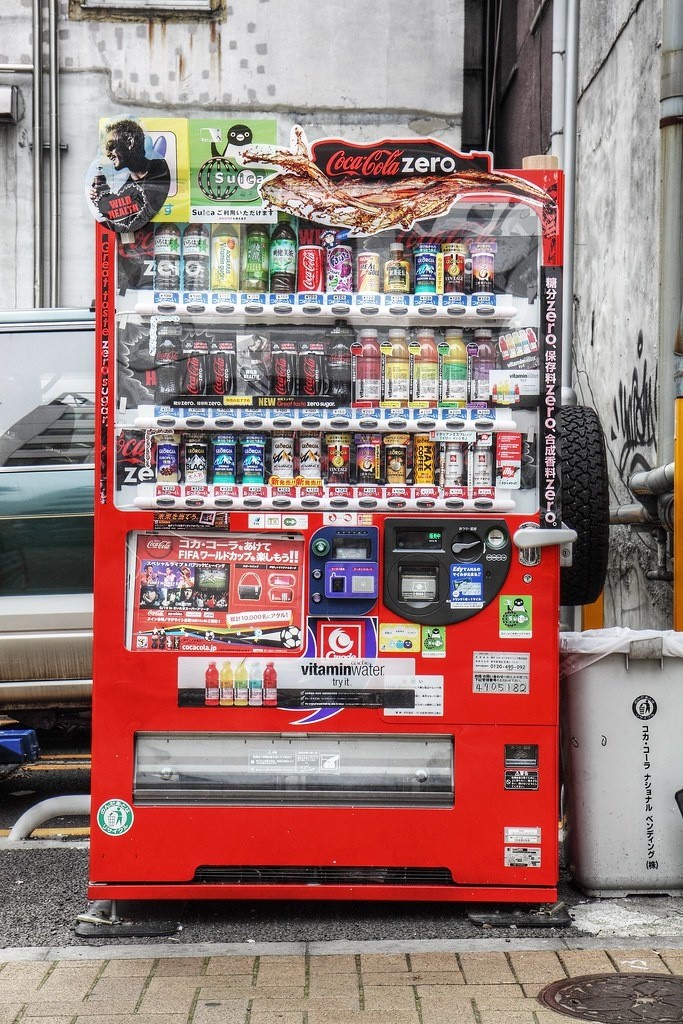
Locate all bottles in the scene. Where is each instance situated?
[384,243,411,293]
[159,627,166,649]
[270,213,297,293]
[155,222,181,290]
[472,329,496,408]
[212,224,239,291]
[474,441,493,503]
[151,626,158,648]
[442,329,468,408]
[263,661,278,706]
[327,319,352,406]
[446,442,463,502]
[220,661,233,706]
[385,329,410,407]
[234,661,248,706]
[183,223,210,290]
[358,329,381,407]
[492,382,520,405]
[205,661,219,706]
[413,329,438,407]
[154,335,180,404]
[94,165,106,195]
[242,223,270,292]
[249,661,262,706]
[499,328,538,360]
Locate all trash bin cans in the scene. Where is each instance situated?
[560,630,683,897]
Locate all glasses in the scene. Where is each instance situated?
[106,140,125,150]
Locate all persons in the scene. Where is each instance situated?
[139,563,228,609]
[88,118,170,230]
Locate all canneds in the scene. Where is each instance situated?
[299,437,322,480]
[271,437,293,480]
[166,636,180,651]
[156,441,265,486]
[413,433,435,484]
[180,348,325,397]
[296,245,380,294]
[414,252,494,296]
[327,443,406,485]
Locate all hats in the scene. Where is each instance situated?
[182,566,191,574]
